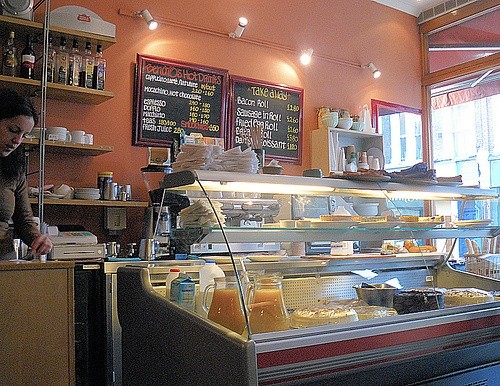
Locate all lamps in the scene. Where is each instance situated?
[133,8,158,31]
[367,62,381,79]
[300,48,314,64]
[227,17,248,39]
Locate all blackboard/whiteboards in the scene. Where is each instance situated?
[132,52,230,151]
[226,73,304,165]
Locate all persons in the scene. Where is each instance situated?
[0,84,53,261]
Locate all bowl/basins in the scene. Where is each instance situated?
[321,112,363,131]
[302,168,323,178]
[263,166,283,175]
[353,284,397,307]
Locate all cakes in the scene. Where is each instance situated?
[441,289,489,308]
[288,299,396,329]
[392,291,445,314]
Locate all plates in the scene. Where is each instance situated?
[74,187,100,200]
[310,219,493,228]
[366,147,385,170]
[198,256,240,263]
[246,255,289,261]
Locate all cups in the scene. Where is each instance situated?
[56,184,72,197]
[295,220,310,227]
[279,219,295,228]
[31,126,94,146]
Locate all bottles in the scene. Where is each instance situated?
[165,269,180,300]
[121,191,130,202]
[2,31,17,77]
[318,106,359,129]
[199,260,225,291]
[19,34,35,79]
[55,36,69,86]
[346,145,380,171]
[69,37,106,90]
[46,35,56,83]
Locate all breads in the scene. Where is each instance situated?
[319,215,446,223]
[465,234,500,275]
[405,240,437,253]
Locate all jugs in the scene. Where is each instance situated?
[202,275,245,336]
[245,271,289,334]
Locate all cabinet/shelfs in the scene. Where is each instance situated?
[115,168,500,386]
[311,128,385,176]
[0,15,150,207]
